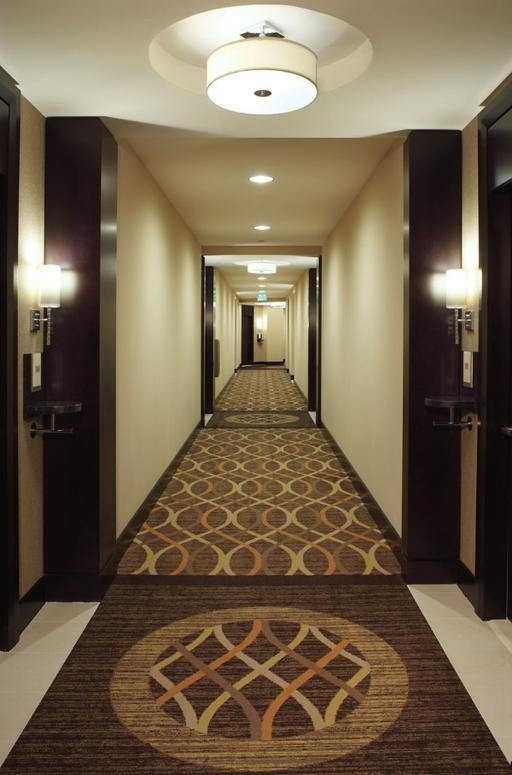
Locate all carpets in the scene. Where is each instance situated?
[0,364,512,775]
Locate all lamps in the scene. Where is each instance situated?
[445,268,477,351]
[30,264,62,347]
[255,319,264,340]
[248,262,277,274]
[206,26,319,119]
